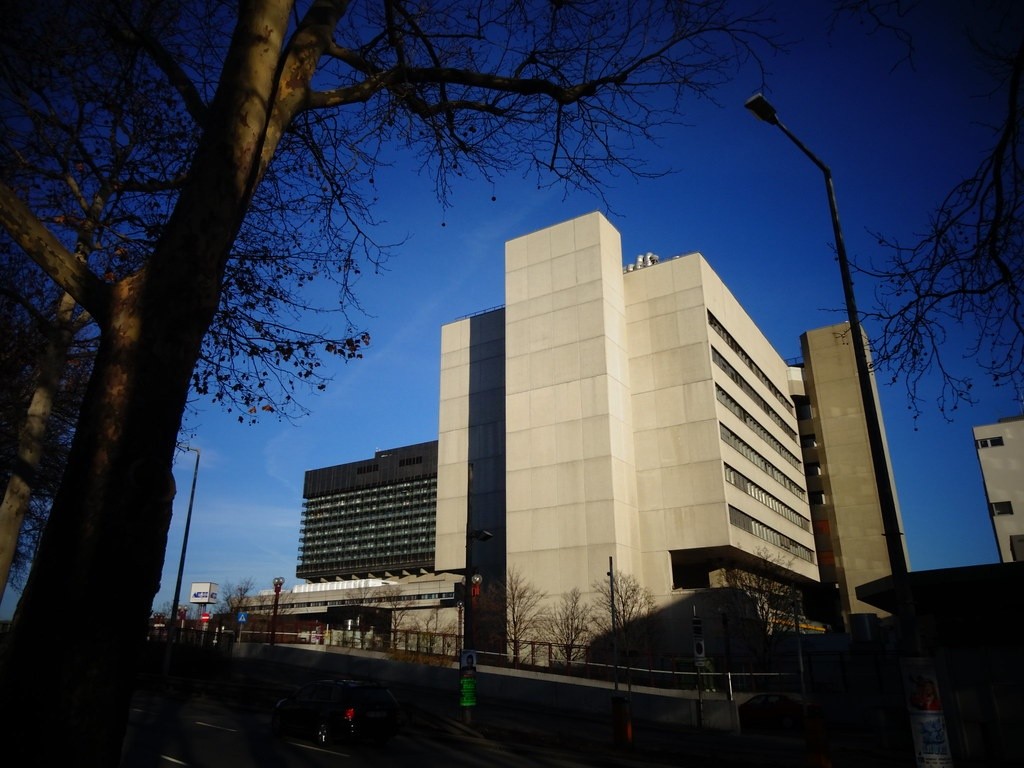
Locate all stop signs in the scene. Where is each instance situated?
[694,638,706,657]
[201,613,210,623]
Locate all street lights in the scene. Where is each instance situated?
[468,529,496,649]
[154,613,166,627]
[744,91,952,768]
[272,577,284,641]
[178,605,189,629]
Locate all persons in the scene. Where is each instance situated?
[362,626,383,651]
[296,619,326,645]
[341,707,360,752]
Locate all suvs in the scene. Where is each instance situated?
[272,679,404,748]
